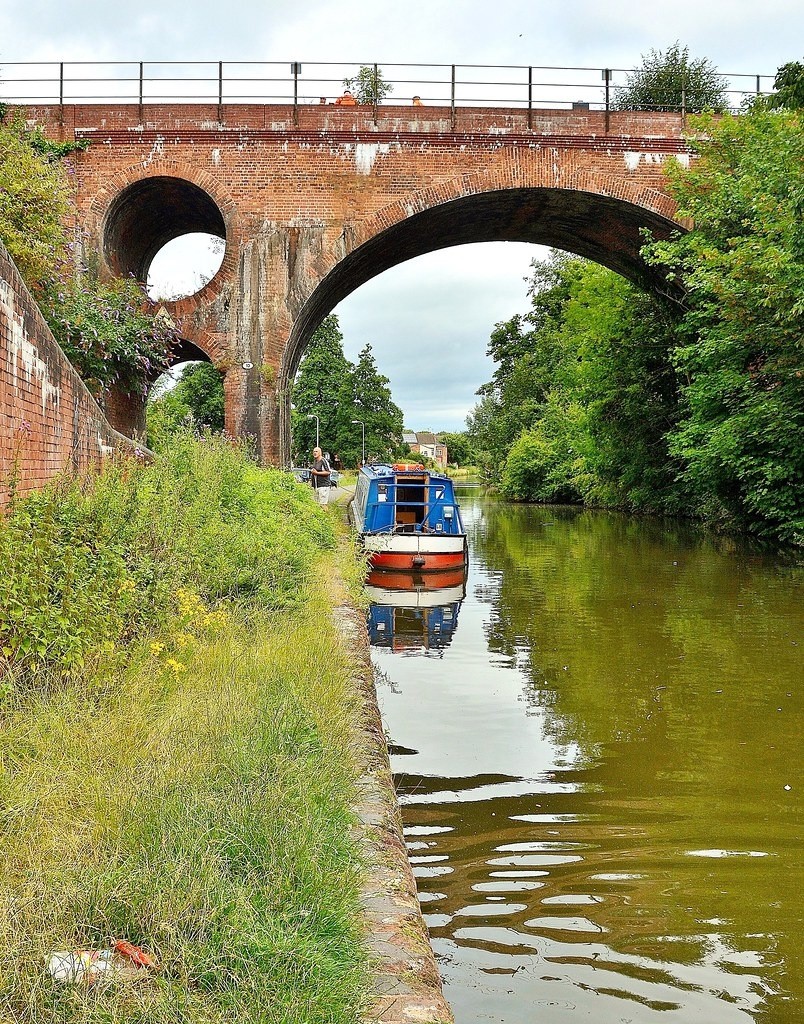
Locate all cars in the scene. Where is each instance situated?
[292,467,312,484]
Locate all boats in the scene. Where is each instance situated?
[365,563,471,652]
[348,463,469,571]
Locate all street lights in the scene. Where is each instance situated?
[307,414,319,447]
[351,420,365,468]
